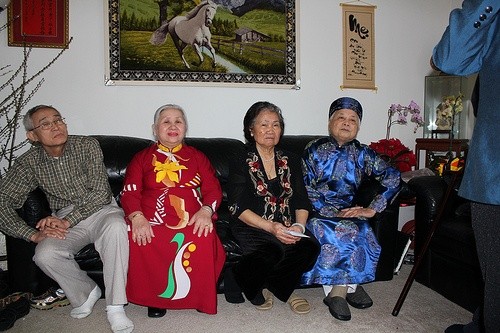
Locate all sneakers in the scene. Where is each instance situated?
[30,286,71,310]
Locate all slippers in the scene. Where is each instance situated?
[255,292,274,310]
[286,294,311,314]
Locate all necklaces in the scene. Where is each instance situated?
[263,153,275,162]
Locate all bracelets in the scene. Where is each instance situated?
[292,222,307,235]
[131,213,143,220]
[201,207,211,215]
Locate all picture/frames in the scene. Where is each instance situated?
[104,0,302,91]
[7,0,69,49]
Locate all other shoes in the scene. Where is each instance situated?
[346,286,373,310]
[147,307,166,319]
[443,323,470,333]
[323,292,351,321]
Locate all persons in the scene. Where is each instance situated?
[0,105,137,332]
[432,0,500,333]
[120,105,227,317]
[223,101,321,305]
[302,97,402,321]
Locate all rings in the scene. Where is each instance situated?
[205,224,210,227]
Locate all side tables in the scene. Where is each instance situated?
[413,138,469,176]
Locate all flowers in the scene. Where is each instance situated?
[438,94,464,131]
[385,101,423,140]
[369,137,417,172]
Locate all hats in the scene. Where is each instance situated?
[328,97,363,122]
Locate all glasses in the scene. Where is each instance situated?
[28,117,66,133]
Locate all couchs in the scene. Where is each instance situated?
[6,136,401,299]
[404,175,484,314]
